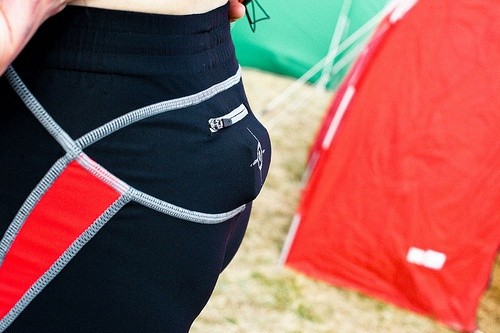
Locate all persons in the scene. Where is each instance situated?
[0,0,272,332]
